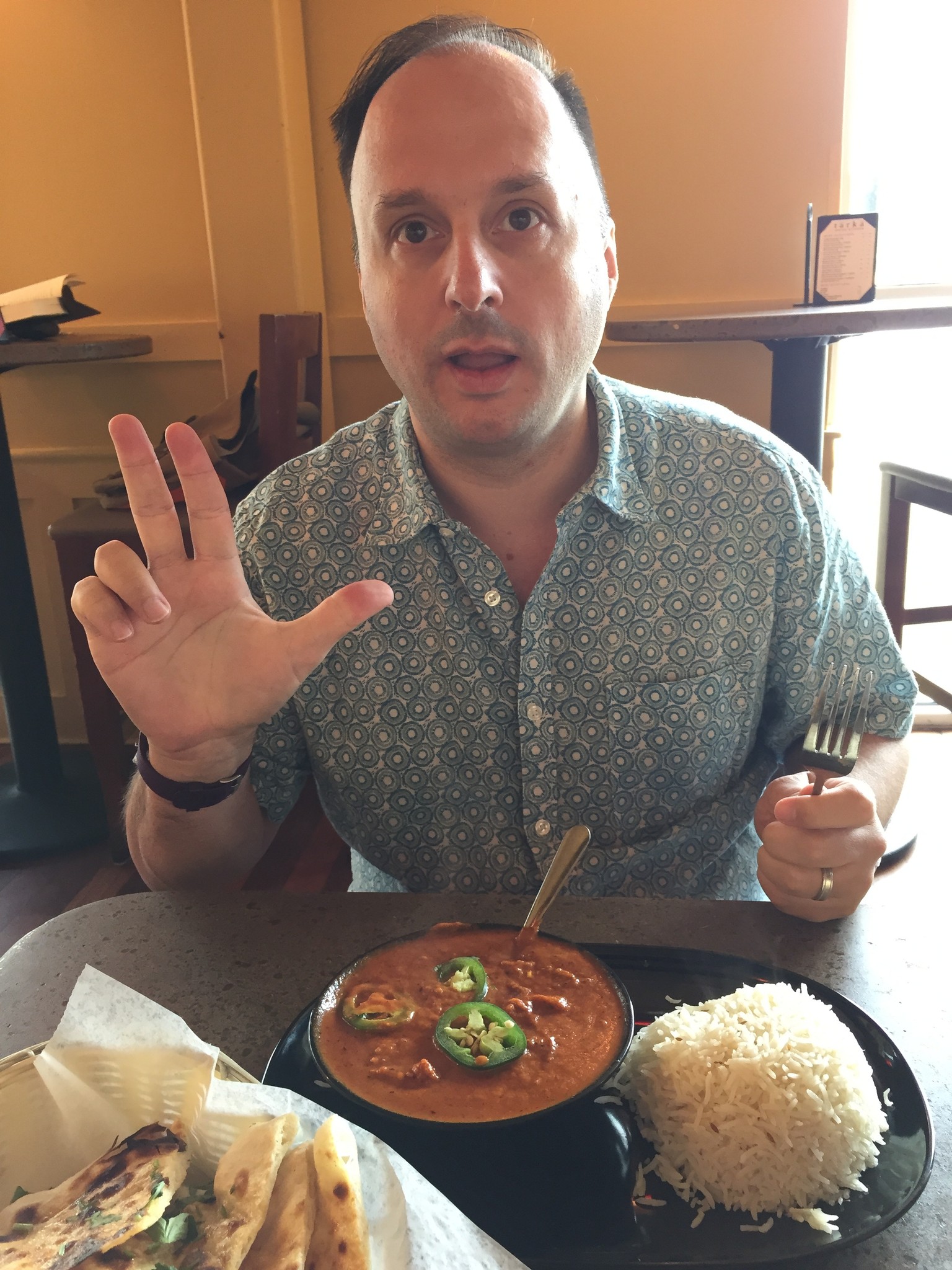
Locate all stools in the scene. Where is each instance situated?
[872,453,952,735]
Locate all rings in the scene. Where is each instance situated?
[811,867,835,904]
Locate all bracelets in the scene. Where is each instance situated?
[124,729,261,810]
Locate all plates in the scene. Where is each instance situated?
[258,943,938,1270]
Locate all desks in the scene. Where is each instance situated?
[0,330,157,877]
[606,298,952,880]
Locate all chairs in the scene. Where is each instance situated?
[48,312,325,866]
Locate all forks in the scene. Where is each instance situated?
[801,662,873,796]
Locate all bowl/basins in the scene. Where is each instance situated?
[303,921,638,1131]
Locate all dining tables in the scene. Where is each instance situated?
[0,889,952,1270]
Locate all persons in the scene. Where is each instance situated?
[66,15,905,924]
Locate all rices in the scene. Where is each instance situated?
[586,977,896,1253]
